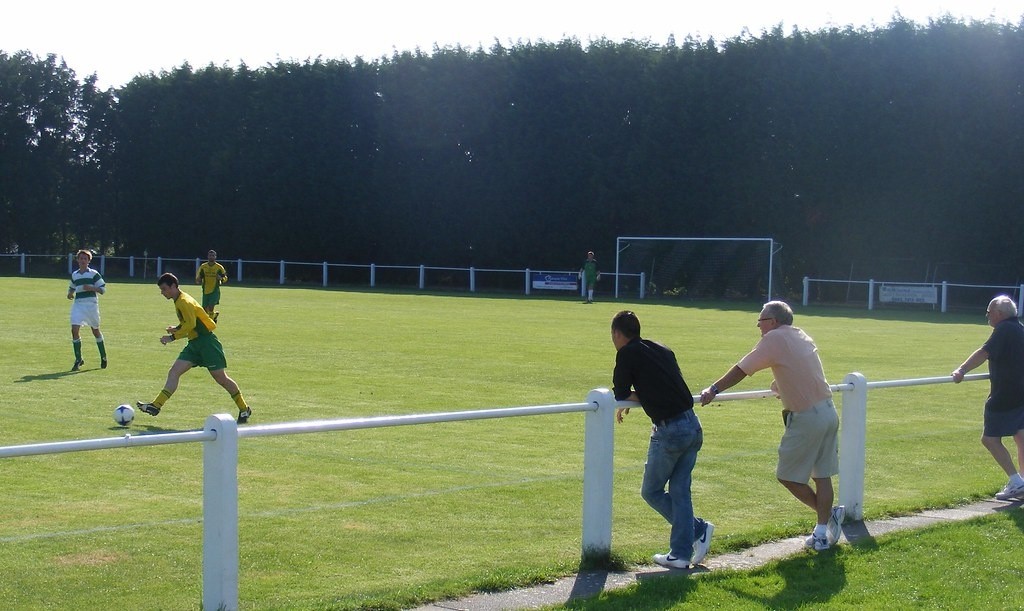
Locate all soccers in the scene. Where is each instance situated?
[114,404,135,426]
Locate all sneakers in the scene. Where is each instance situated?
[136,400,161,416]
[653,550,690,569]
[237,406,252,425]
[692,523,715,565]
[828,505,846,546]
[805,532,830,551]
[995,480,1024,500]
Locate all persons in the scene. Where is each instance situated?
[578,251,601,304]
[195,250,228,323]
[952,295,1024,499]
[66,249,107,372]
[698,300,846,552]
[610,309,714,570]
[135,272,252,426]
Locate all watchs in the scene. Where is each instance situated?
[710,384,719,395]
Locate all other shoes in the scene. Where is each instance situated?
[213,311,219,324]
[101,354,107,369]
[72,359,84,371]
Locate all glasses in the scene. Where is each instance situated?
[757,317,773,324]
